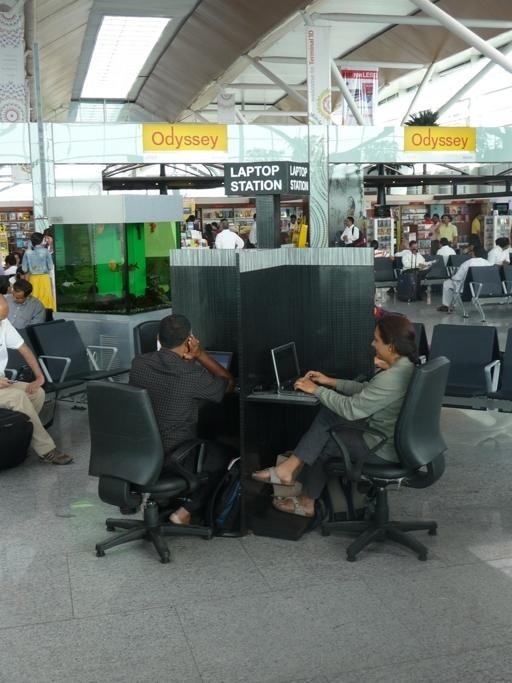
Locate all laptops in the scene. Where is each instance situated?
[194,351,233,370]
[271,341,332,398]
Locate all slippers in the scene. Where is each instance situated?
[248,467,297,488]
[270,492,315,518]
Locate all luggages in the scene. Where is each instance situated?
[396,248,424,303]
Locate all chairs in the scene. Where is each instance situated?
[410,323,512,409]
[87,379,213,563]
[0,319,130,470]
[322,355,451,562]
[374,255,512,322]
[133,320,162,356]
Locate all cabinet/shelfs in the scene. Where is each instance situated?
[394,204,480,250]
[180,208,303,241]
[0,208,35,255]
[484,215,512,251]
[367,217,394,255]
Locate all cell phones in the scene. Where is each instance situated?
[7,380,17,383]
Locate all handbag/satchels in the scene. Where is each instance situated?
[351,225,366,247]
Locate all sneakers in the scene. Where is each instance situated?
[436,305,453,313]
[385,288,396,296]
[38,450,76,467]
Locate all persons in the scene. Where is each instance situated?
[128,313,234,524]
[183,213,298,249]
[248,312,420,519]
[341,210,512,312]
[0,228,55,329]
[0,293,73,465]
